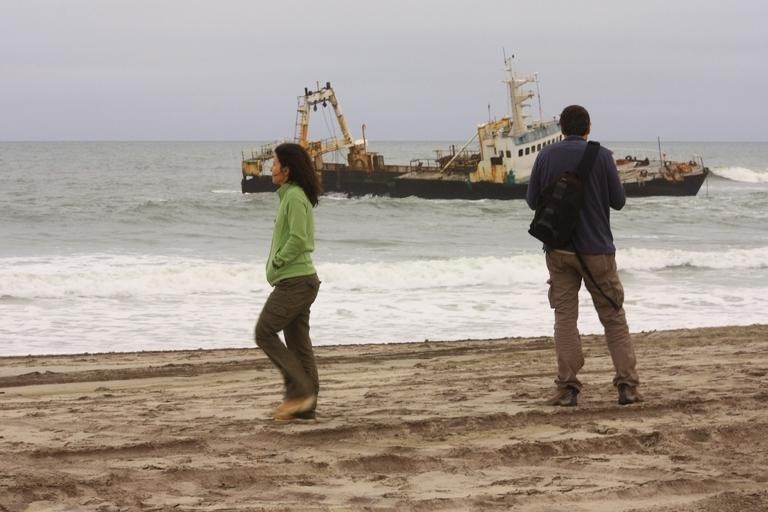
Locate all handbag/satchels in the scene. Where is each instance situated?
[528,177,569,245]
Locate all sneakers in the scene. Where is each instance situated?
[618,383,646,404]
[543,385,580,407]
[272,394,317,422]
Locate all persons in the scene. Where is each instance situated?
[525,105,644,407]
[251,141,325,418]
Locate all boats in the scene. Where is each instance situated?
[241,47,711,201]
